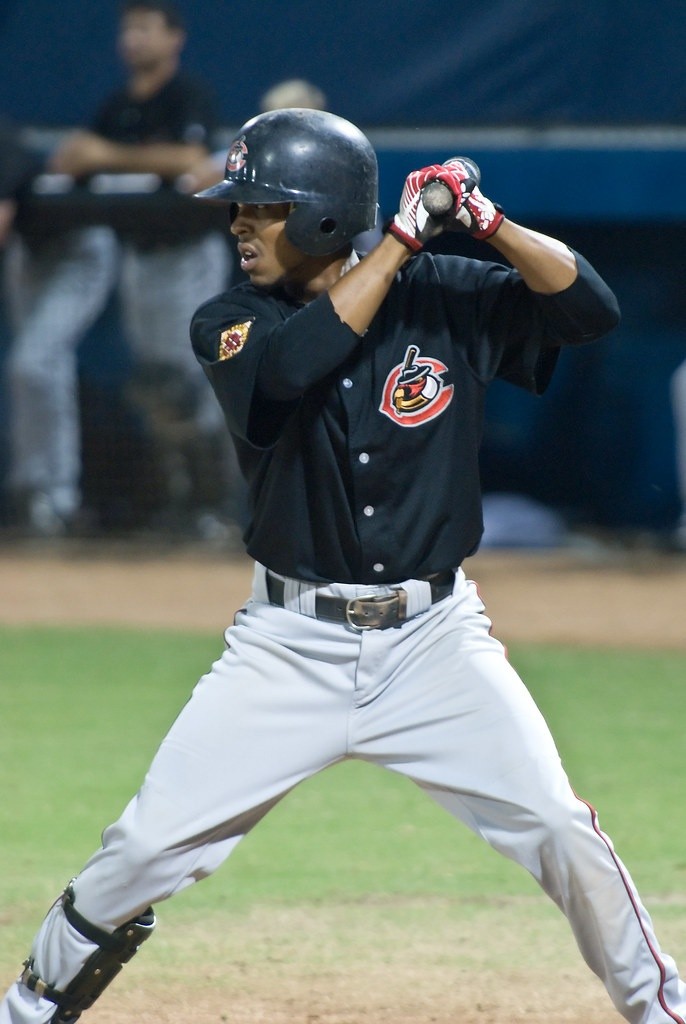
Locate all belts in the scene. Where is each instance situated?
[265,569,454,631]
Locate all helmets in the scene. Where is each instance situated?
[193,107,377,256]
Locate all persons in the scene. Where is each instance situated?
[0,107,686,1024]
[0,0,323,545]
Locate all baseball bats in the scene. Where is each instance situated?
[422,157,481,216]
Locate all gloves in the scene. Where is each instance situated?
[387,161,505,252]
